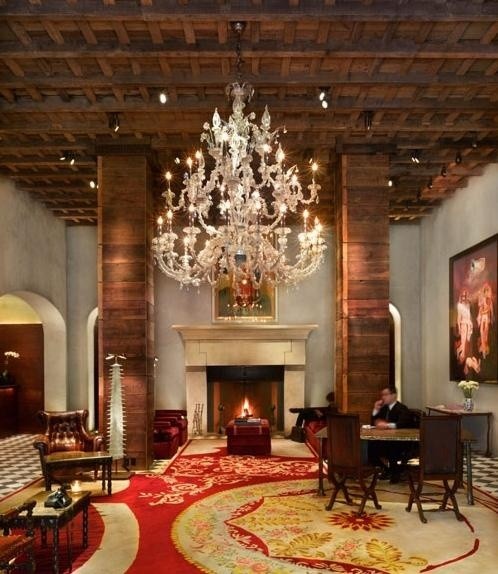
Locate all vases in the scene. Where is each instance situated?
[464,397,474,411]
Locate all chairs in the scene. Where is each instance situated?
[155,409,188,446]
[0,502,34,574]
[323,410,381,517]
[402,413,461,524]
[32,409,104,480]
[153,416,180,459]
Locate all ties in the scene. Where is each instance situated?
[385,405,390,422]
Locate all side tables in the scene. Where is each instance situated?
[44,450,112,495]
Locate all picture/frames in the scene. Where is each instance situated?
[449,233,497,385]
[211,229,277,325]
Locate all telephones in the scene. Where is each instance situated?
[44,486,72,508]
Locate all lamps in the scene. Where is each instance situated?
[58,151,80,165]
[104,111,120,134]
[89,180,98,190]
[318,88,330,109]
[427,137,478,190]
[151,21,325,306]
[410,149,423,163]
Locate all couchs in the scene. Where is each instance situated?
[304,420,329,472]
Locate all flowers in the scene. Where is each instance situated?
[458,380,480,397]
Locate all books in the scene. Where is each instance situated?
[233,416,261,425]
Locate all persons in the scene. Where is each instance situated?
[455,288,474,361]
[370,386,419,485]
[284,391,334,442]
[477,284,495,359]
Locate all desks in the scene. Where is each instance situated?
[426,405,492,456]
[3,489,92,574]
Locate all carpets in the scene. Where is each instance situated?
[397,451,498,500]
[0,432,43,499]
